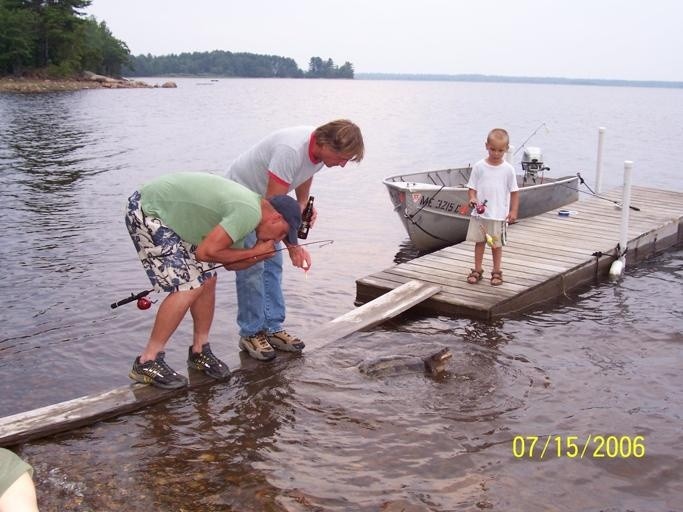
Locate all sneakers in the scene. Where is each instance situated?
[239,331,276,361]
[188,342,229,379]
[266,329,305,352]
[130,352,187,389]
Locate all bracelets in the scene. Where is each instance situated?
[254,256,258,261]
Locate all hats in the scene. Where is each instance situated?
[267,195,303,243]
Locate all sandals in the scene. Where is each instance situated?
[491,271,502,285]
[467,269,483,284]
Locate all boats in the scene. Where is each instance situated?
[378,123,582,252]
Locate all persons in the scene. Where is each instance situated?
[124,173,302,391]
[465,129,519,286]
[223,118,365,361]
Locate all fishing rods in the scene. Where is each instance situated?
[111,239,333,308]
[513,121,639,212]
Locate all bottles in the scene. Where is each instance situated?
[298,196,314,240]
